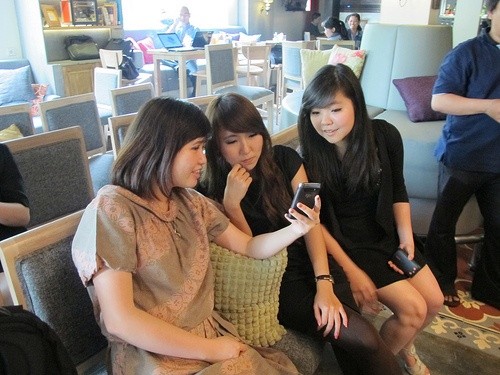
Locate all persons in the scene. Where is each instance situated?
[194,92,412,375]
[305,12,327,37]
[0,143,33,273]
[297,63,446,375]
[347,13,363,44]
[158,5,207,97]
[69,97,321,375]
[320,15,349,41]
[424,0,500,308]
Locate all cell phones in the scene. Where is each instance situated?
[288,183,321,219]
[391,250,421,277]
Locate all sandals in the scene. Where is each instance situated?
[440,285,461,307]
[400,344,430,375]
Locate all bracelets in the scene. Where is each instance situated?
[315,275,335,283]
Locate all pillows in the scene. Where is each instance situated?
[391,75,449,122]
[0,65,36,104]
[300,48,334,88]
[326,44,367,78]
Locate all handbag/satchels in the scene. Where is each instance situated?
[103,38,132,55]
[0,305,78,375]
[65,35,99,59]
[119,56,139,79]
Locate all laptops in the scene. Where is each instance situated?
[157,33,196,52]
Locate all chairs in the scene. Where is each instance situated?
[0,30,373,375]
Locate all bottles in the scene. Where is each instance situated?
[303,31,311,41]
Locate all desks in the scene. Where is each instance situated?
[147,47,244,100]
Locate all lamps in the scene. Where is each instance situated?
[261,0,273,16]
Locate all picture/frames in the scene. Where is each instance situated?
[41,0,116,28]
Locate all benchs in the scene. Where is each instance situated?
[126,25,252,98]
